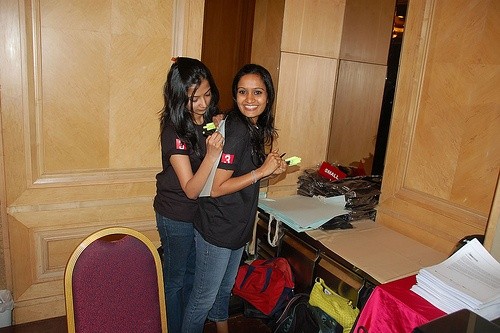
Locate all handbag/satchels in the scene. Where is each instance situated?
[232,257,361,333]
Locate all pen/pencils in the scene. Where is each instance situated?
[203,131,212,136]
[280,152,287,158]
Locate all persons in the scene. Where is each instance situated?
[181,64,274,333]
[151,56,225,333]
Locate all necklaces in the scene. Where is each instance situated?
[191,115,206,134]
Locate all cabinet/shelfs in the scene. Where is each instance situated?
[256,207,450,321]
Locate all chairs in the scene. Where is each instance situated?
[63,226,168,333]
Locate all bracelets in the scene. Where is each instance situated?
[251,170,257,184]
